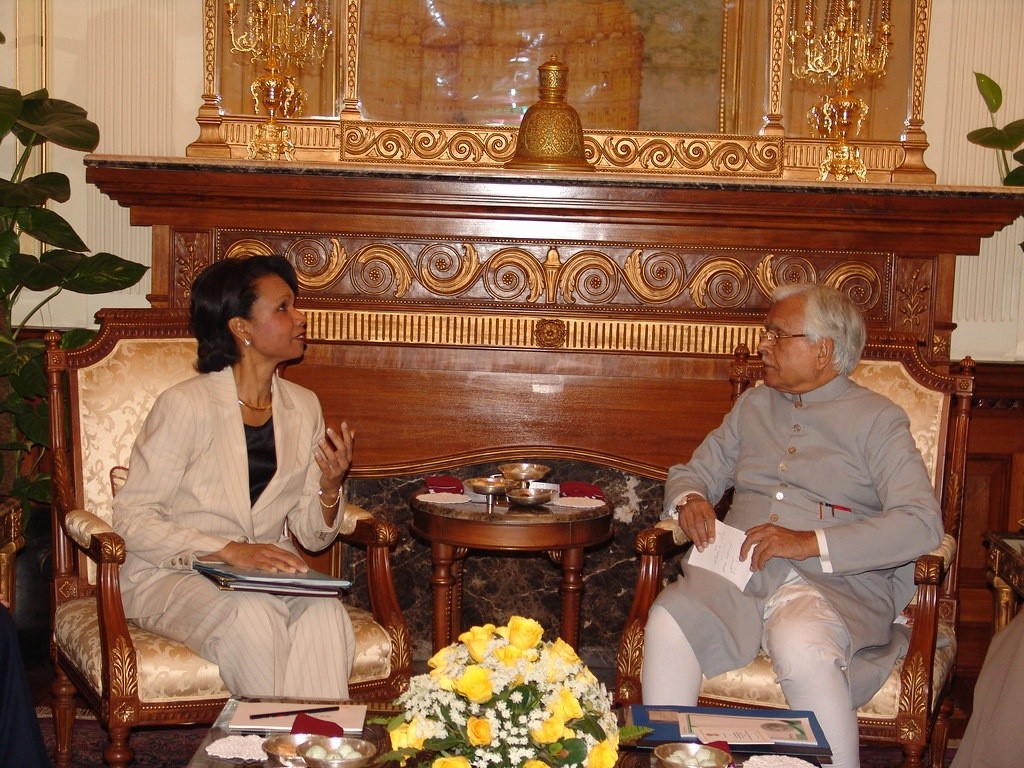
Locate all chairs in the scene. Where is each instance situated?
[35,308,413,768]
[621,339,977,768]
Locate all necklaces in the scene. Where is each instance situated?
[239,399,272,415]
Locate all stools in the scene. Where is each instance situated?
[982,531,1024,638]
[0,495,26,622]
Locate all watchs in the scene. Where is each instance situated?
[673,493,708,513]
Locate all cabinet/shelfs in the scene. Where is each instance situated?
[83,152,1024,482]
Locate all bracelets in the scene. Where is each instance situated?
[319,485,343,508]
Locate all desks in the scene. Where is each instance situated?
[188,693,822,768]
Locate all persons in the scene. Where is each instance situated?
[641,280,948,768]
[111,254,358,701]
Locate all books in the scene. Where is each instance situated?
[192,560,353,599]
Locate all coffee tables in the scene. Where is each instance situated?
[412,484,616,655]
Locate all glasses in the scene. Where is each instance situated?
[759,327,810,345]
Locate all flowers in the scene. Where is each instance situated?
[366,615,655,768]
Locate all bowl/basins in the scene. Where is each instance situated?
[298,737,378,768]
[506,489,557,505]
[262,733,328,768]
[654,742,733,768]
